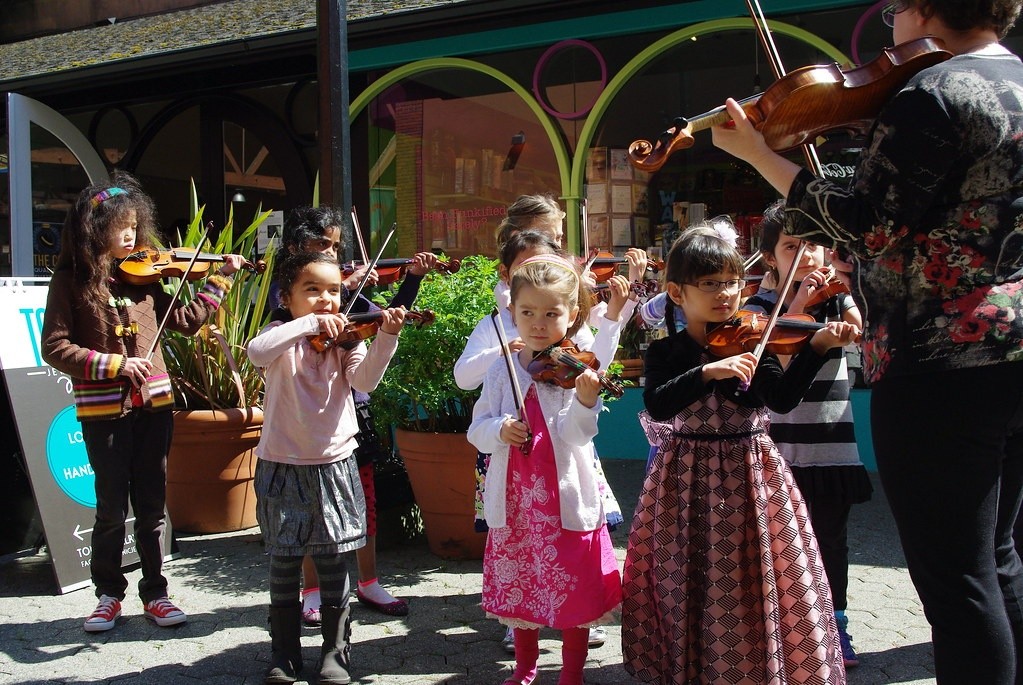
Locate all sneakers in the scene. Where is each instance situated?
[84,595,121,632]
[144,597,186,626]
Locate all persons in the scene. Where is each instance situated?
[741,201,874,669]
[494,195,647,335]
[268,204,438,626]
[41,173,246,631]
[453,231,630,652]
[711,0,1023,685]
[621,216,859,685]
[248,251,405,685]
[466,254,621,685]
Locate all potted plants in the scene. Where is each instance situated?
[354,255,635,560]
[143,176,278,534]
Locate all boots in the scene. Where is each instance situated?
[317,606,351,684]
[268,604,303,683]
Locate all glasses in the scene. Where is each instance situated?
[882,0,908,27]
[681,280,747,292]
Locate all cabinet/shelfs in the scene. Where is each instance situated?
[428,195,514,255]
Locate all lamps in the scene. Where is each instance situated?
[512,131,526,143]
[232,189,245,202]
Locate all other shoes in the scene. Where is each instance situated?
[502,627,517,650]
[357,591,408,615]
[589,626,607,644]
[836,616,859,661]
[302,598,322,629]
[504,669,539,685]
